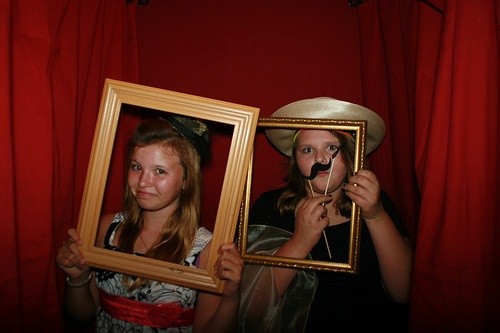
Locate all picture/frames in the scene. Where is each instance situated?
[238,118,367,274]
[74,78,260,294]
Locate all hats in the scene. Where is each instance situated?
[265,97,385,157]
[159,115,209,157]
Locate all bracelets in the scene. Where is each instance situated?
[65,274,93,288]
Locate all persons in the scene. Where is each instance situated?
[235,95,411,333]
[55,112,243,333]
